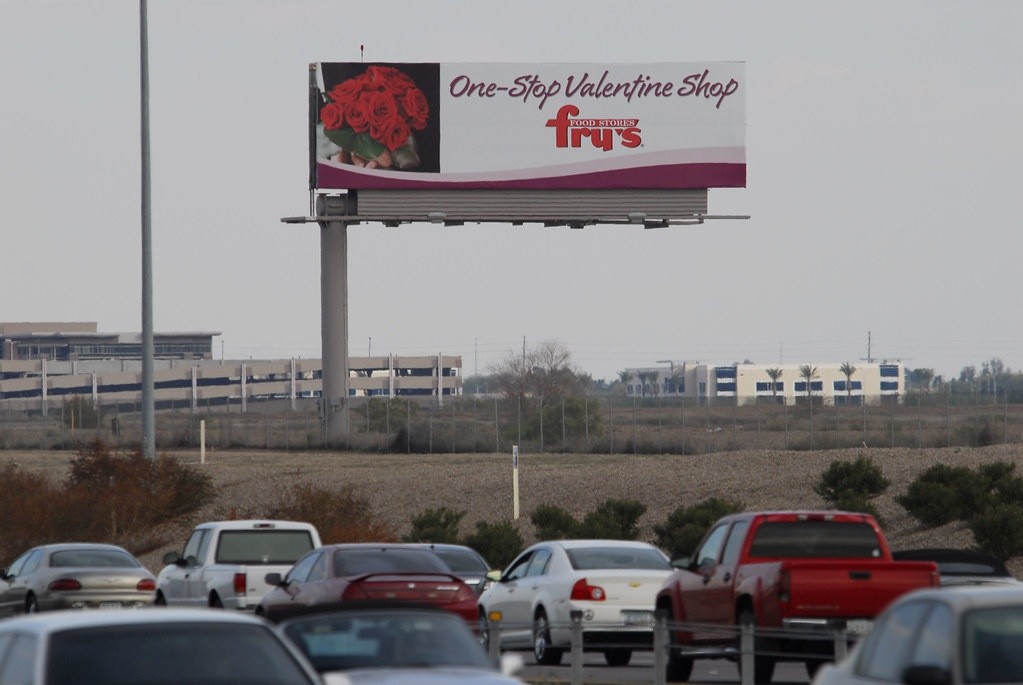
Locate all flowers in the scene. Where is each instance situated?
[318,64,431,165]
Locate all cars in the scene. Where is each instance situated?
[810,583,1022,684]
[0,611,328,684]
[892,547,1020,586]
[0,540,157,616]
[478,537,681,665]
[273,606,527,685]
[256,541,482,636]
[404,543,497,602]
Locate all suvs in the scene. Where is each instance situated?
[159,518,331,616]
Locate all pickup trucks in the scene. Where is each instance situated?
[649,507,941,685]
